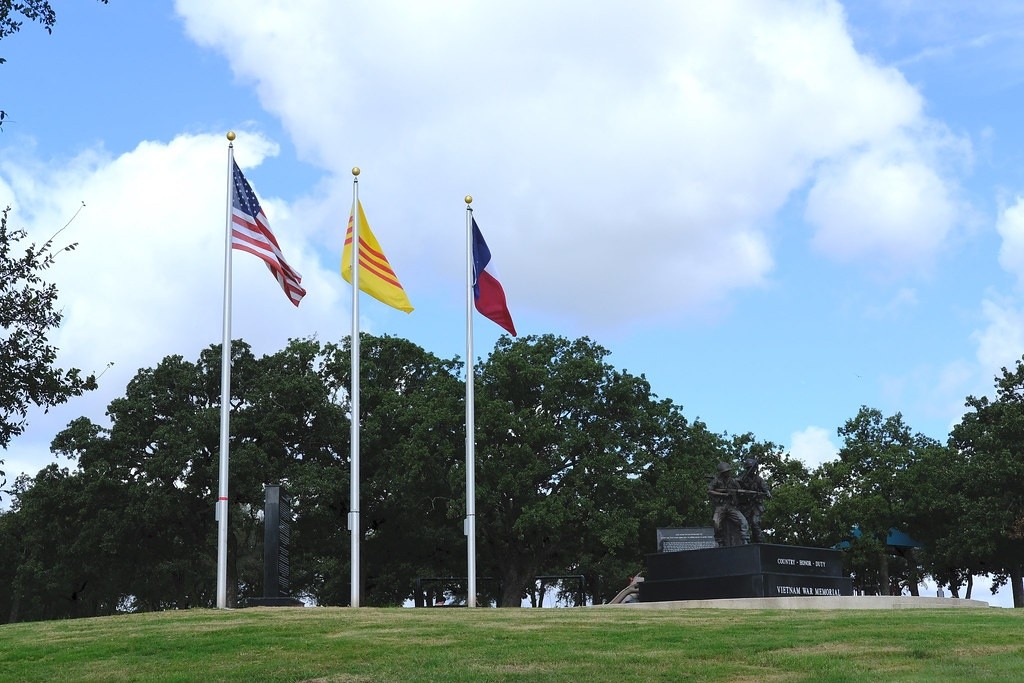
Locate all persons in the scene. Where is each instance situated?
[736,458,771,542]
[709,461,751,545]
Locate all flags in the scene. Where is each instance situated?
[231,158,307,308]
[340,200,413,313]
[472,217,517,336]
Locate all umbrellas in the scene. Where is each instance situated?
[835,522,924,548]
